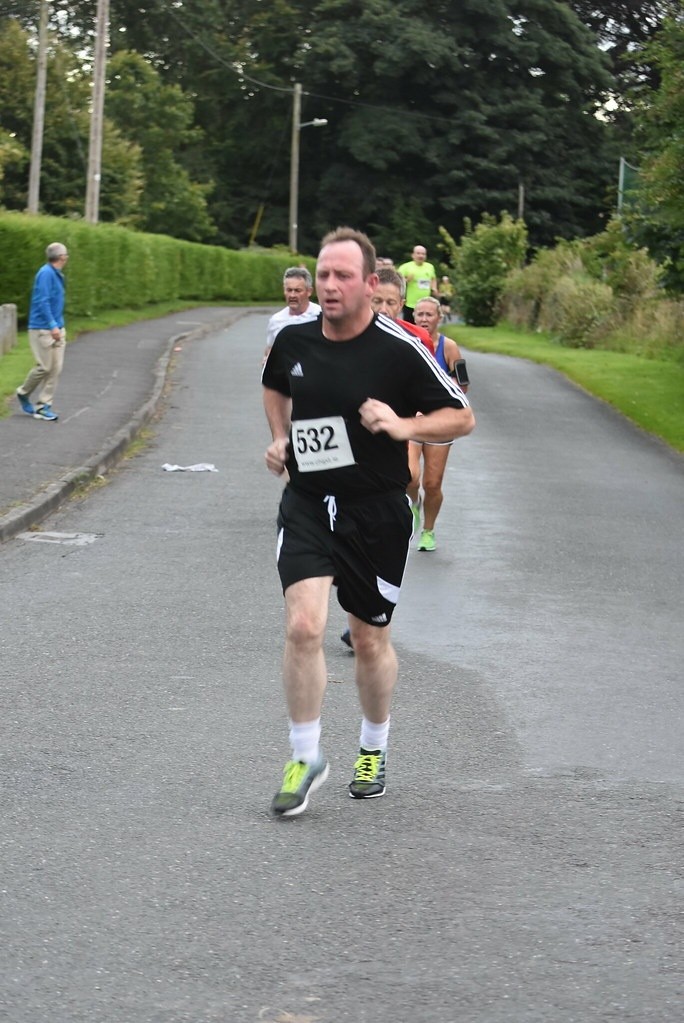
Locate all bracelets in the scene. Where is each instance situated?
[49,322,57,327]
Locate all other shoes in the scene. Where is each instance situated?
[340,628,352,646]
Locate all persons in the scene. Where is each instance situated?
[258,265,323,434]
[340,257,439,655]
[16,242,69,421]
[397,245,440,326]
[261,226,477,819]
[406,296,470,552]
[439,275,455,324]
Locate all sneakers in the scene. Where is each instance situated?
[17,393,35,414]
[417,529,435,551]
[271,750,329,816]
[33,405,57,420]
[409,493,422,529]
[348,746,387,798]
